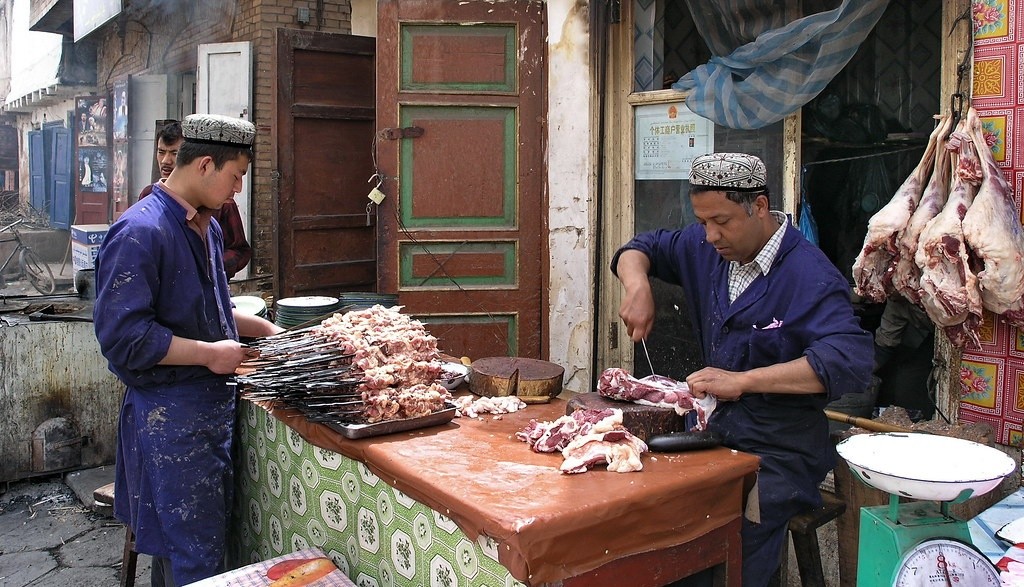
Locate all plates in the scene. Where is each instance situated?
[275,296,339,330]
[339,292,399,311]
[229,296,269,320]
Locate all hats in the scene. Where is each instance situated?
[181,114,256,148]
[689,153,767,193]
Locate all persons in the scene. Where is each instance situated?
[94,113,288,587]
[607,151,875,587]
[77,88,128,203]
[872,299,936,422]
[138,121,255,283]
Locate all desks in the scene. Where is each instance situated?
[184,547,358,587]
[232,354,762,587]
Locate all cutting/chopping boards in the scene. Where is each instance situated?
[566,392,685,442]
[470,357,566,400]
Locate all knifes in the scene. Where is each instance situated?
[461,355,472,384]
[641,337,657,381]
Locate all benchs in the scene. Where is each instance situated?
[773,495,848,587]
[94,483,142,587]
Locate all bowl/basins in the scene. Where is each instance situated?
[434,362,466,390]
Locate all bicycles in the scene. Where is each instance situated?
[0,214,57,296]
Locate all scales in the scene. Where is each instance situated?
[835,431,1017,587]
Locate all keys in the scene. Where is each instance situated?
[367,201,374,207]
